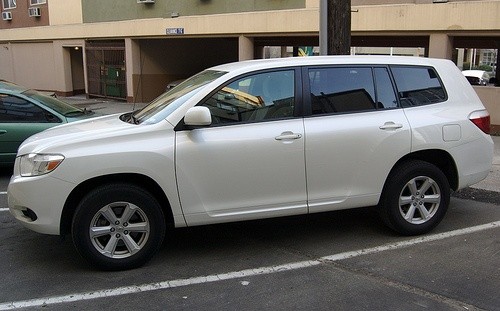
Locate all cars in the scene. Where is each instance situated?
[0,78,108,178]
[462,70,490,86]
[485,61,496,70]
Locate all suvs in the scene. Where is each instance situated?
[7,54,494,273]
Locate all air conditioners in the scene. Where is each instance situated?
[2,12,12,20]
[137,0,155,4]
[28,8,41,17]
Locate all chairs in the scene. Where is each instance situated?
[249,74,293,120]
[243,77,262,111]
